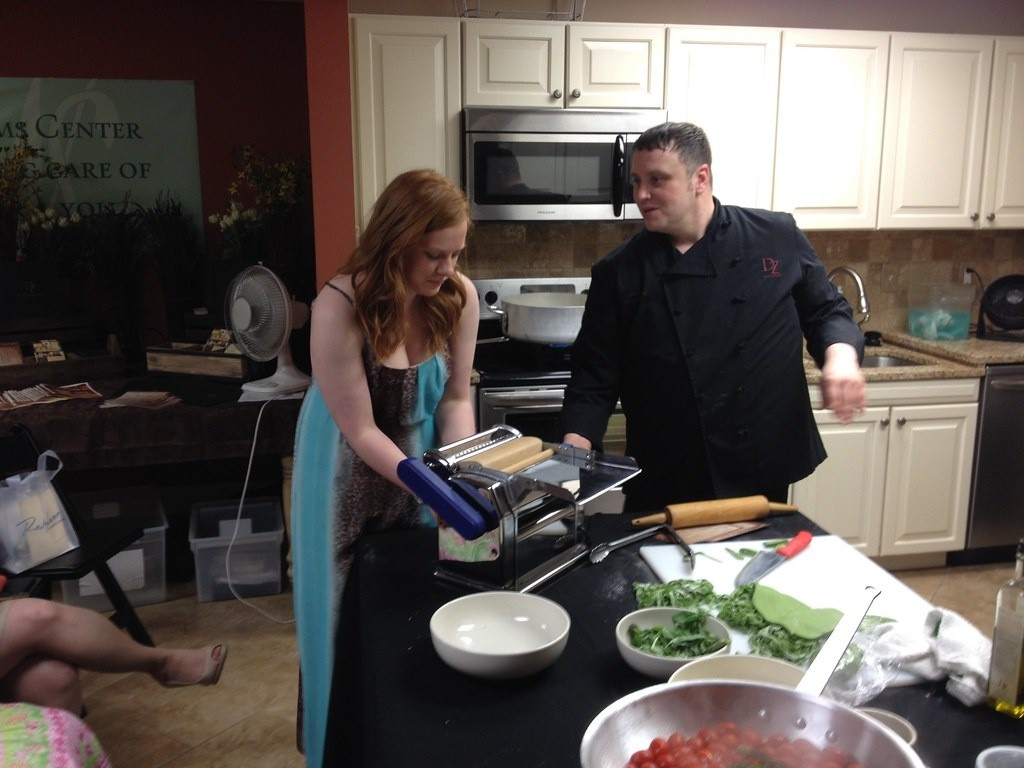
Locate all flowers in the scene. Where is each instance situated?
[205,142,309,259]
[0,135,53,213]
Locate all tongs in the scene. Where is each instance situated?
[589,521,696,571]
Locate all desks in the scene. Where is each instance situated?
[326,470,1024,768]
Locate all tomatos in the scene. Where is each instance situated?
[624,722,867,768]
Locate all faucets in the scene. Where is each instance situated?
[828,267,870,314]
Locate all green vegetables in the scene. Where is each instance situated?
[628,580,821,664]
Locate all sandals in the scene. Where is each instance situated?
[150,644,227,688]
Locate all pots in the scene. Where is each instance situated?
[487,292,588,345]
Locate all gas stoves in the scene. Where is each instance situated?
[472,276,592,387]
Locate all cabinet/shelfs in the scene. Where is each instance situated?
[468,19,665,110]
[665,24,891,236]
[348,15,464,244]
[880,32,1024,232]
[788,382,982,575]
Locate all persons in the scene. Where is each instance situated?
[293,170,481,764]
[0,595,229,718]
[561,123,865,512]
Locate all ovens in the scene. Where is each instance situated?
[476,387,628,462]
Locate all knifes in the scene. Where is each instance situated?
[734,530,813,588]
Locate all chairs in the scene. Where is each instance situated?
[0,422,153,718]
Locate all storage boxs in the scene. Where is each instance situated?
[187,497,285,603]
[60,502,170,612]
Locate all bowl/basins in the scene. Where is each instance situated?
[429,590,572,680]
[579,678,927,768]
[974,744,1024,768]
[859,707,918,746]
[615,606,732,679]
[667,654,806,689]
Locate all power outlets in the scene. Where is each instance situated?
[964,268,973,286]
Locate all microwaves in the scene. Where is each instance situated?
[462,104,669,224]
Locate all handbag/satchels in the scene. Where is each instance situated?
[0,450,80,574]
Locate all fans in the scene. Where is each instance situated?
[228,263,314,395]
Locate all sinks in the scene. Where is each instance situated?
[858,349,938,368]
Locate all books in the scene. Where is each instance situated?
[203,328,233,355]
[0,381,106,411]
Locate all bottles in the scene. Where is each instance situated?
[985,541,1024,718]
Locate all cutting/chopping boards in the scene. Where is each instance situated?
[639,534,935,688]
